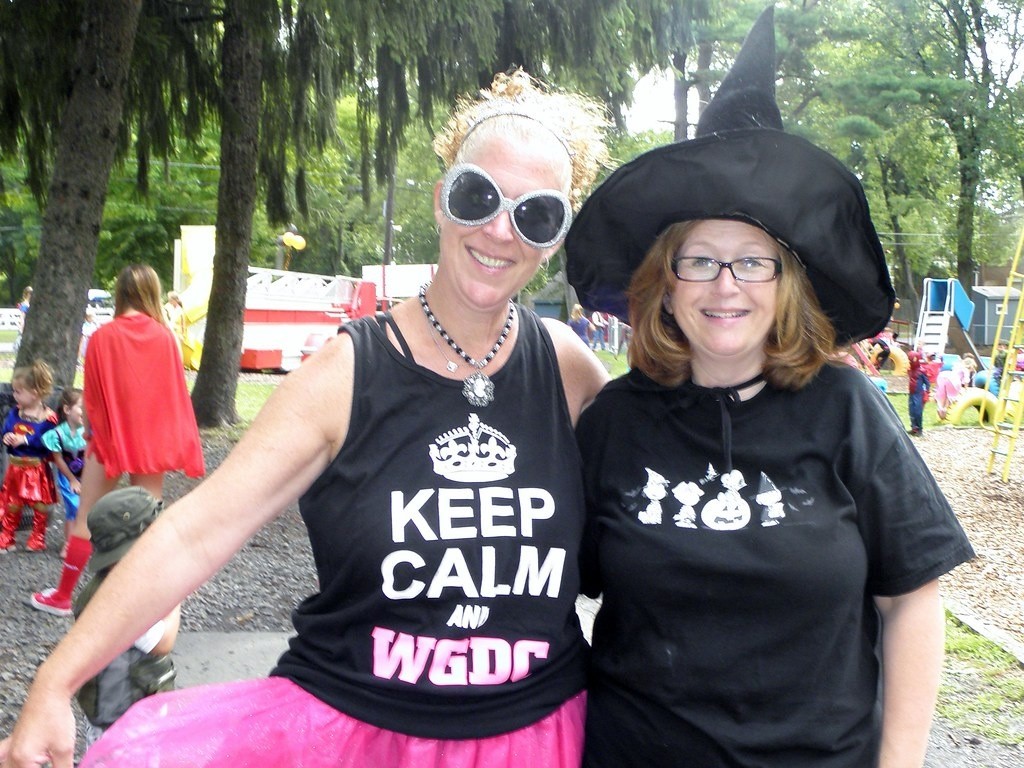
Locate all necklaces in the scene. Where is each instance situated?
[417,280,515,407]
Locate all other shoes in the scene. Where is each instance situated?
[59,541,69,559]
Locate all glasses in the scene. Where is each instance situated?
[672,253,782,282]
[438,163,573,247]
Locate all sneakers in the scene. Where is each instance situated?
[30,588,71,616]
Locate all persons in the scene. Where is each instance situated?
[904,351,931,438]
[0,360,59,554]
[70,484,181,734]
[40,386,87,560]
[566,303,596,349]
[591,311,609,351]
[994,341,1009,368]
[164,290,186,342]
[572,6,976,768]
[0,69,617,768]
[31,265,208,616]
[82,313,97,358]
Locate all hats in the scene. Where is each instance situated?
[565,6,896,347]
[87,486,164,574]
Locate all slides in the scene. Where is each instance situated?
[947,314,988,372]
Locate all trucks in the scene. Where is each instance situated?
[234,264,378,374]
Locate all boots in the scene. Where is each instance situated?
[0,508,23,553]
[25,509,47,552]
